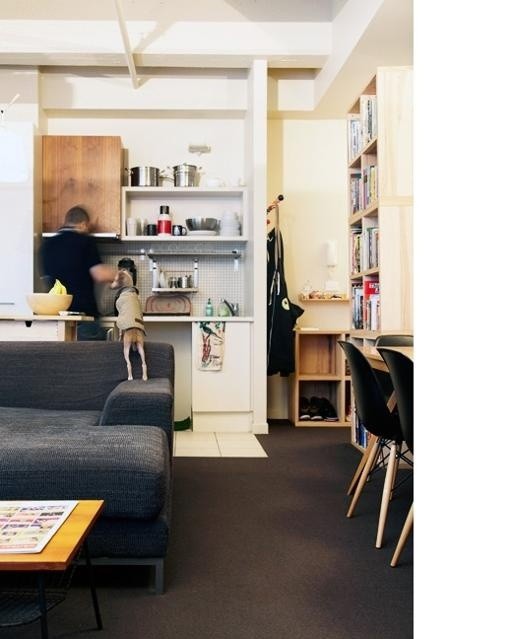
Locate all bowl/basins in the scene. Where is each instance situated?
[159,272,167,288]
[25,293,73,315]
[219,210,240,236]
[186,218,218,230]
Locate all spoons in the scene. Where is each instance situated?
[58,311,85,315]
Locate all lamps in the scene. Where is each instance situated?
[324,254,340,272]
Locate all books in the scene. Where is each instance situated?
[363,163,378,208]
[363,280,380,330]
[348,118,360,162]
[363,226,379,269]
[350,230,362,276]
[349,170,361,214]
[361,96,375,141]
[351,284,363,330]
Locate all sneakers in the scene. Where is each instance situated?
[309,396,323,421]
[299,396,311,421]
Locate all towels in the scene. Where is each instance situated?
[197,322,225,372]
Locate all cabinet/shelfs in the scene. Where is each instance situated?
[192,322,253,431]
[289,329,353,428]
[346,73,413,470]
[42,135,123,234]
[120,186,247,243]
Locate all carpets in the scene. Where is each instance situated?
[1,590,64,626]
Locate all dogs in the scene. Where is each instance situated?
[109,267,148,381]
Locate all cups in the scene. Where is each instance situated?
[172,225,187,235]
[144,224,156,236]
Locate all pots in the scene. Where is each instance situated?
[166,162,202,186]
[125,166,165,186]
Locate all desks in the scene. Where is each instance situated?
[346,345,414,497]
[0,313,94,340]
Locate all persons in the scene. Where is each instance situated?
[41,207,119,341]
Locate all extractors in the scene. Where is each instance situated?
[43,232,120,244]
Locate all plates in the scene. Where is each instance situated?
[187,230,217,235]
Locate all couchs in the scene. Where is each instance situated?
[1,341,175,596]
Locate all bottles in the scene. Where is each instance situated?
[127,218,137,236]
[324,264,340,299]
[217,297,230,316]
[156,202,172,236]
[206,298,212,316]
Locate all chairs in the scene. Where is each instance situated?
[337,340,404,548]
[377,347,412,567]
[367,335,413,496]
